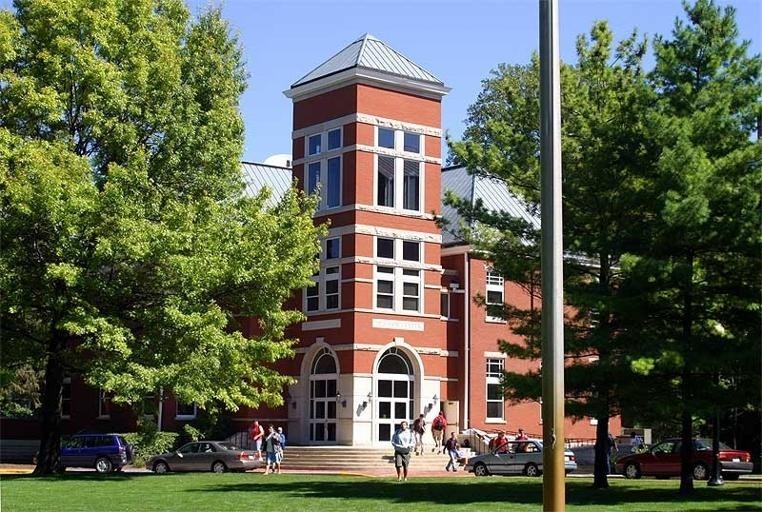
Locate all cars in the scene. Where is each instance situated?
[464,439,579,478]
[615,435,645,455]
[146,441,261,474]
[616,438,755,482]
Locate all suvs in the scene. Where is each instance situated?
[33,432,134,473]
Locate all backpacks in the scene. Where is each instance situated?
[434,420,443,431]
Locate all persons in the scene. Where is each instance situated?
[628,431,644,455]
[246,418,286,476]
[607,433,619,474]
[391,408,529,483]
